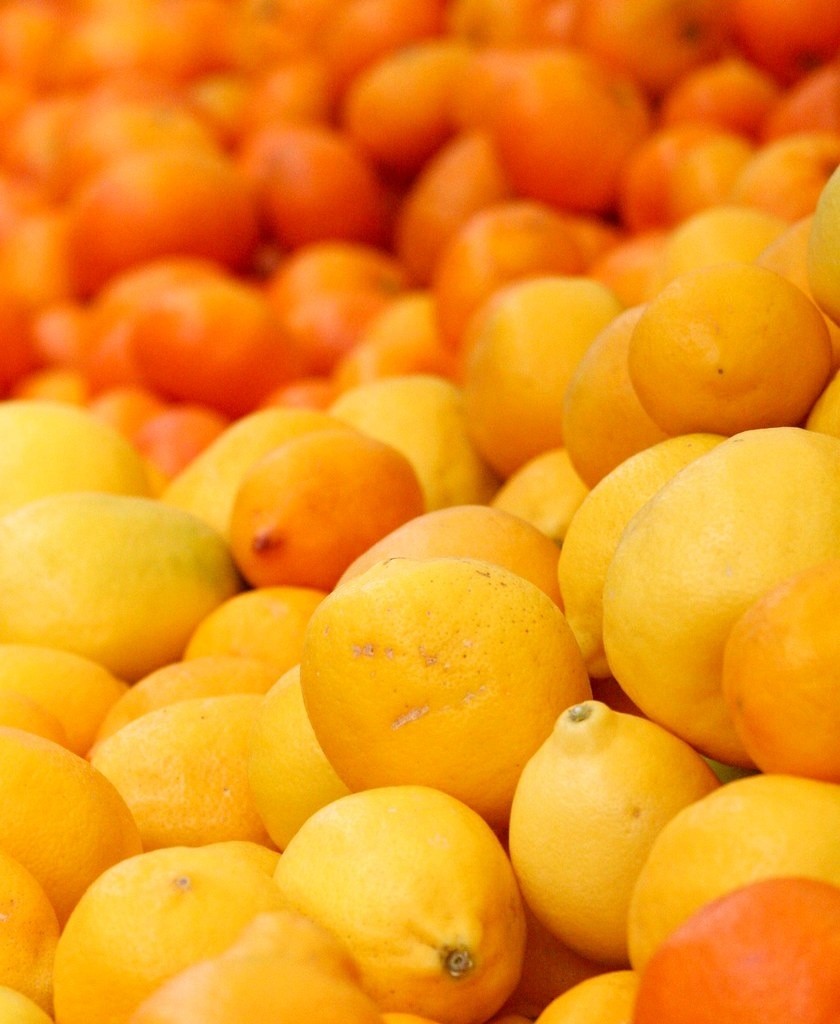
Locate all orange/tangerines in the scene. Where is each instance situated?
[0,0,840,465]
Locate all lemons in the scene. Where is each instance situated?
[0,157,839,1024]
